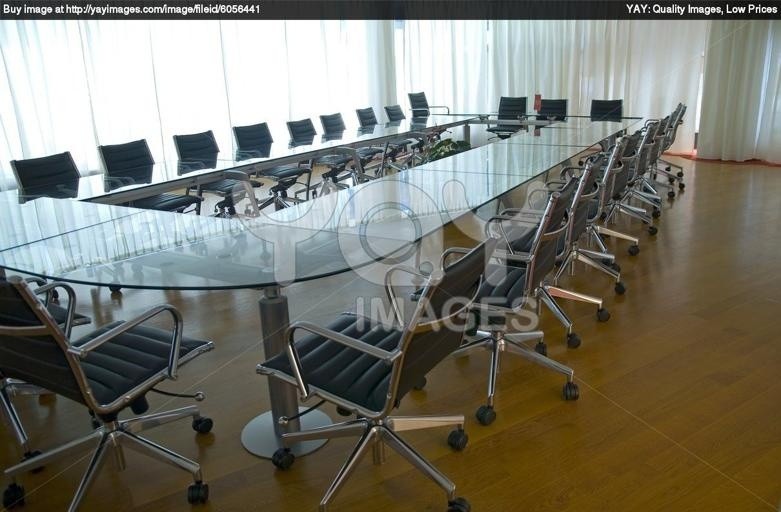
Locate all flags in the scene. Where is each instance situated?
[533,94,544,111]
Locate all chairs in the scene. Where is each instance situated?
[0,92,686,511]
